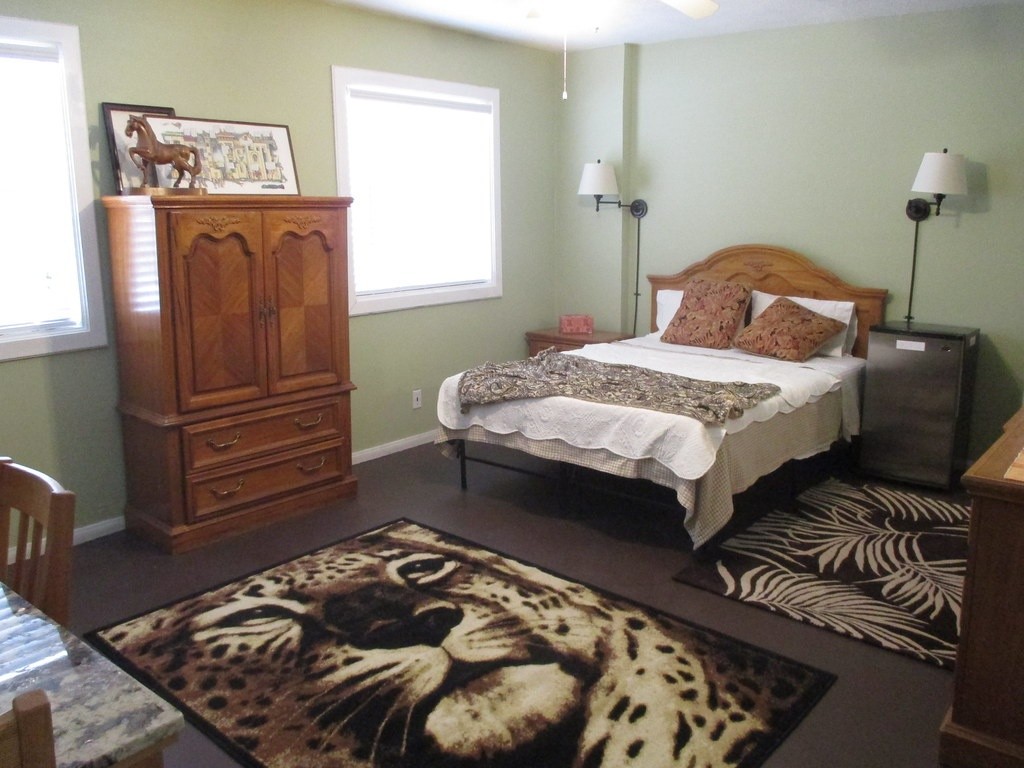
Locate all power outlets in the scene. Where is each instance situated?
[412,389,424,411]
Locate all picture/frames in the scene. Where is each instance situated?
[101,101,177,197]
[143,113,302,197]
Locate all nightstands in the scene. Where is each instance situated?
[525,326,635,362]
[842,320,982,507]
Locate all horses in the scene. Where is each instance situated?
[125,113,203,189]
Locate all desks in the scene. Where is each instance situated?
[936,403,1024,768]
[0,578,188,768]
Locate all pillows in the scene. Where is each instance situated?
[731,294,846,363]
[659,278,754,350]
[750,288,860,358]
[654,287,745,340]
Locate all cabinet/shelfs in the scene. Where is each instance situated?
[101,194,356,558]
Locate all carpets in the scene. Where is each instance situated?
[670,466,972,673]
[77,514,840,768]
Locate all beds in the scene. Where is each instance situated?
[428,246,890,558]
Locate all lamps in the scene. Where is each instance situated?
[904,146,970,223]
[576,157,647,220]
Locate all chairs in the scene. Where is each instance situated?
[0,454,75,631]
[0,688,58,768]
